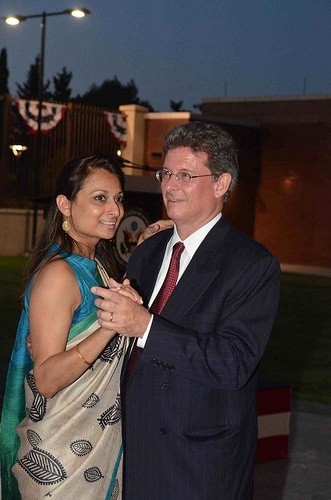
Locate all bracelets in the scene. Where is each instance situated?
[76,344,92,366]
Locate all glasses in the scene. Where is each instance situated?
[155,170,213,185]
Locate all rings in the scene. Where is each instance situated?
[109,312,114,322]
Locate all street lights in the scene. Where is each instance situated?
[0,8,92,250]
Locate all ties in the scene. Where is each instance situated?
[125,242,185,380]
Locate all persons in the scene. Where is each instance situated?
[0,154,174,500]
[90,120,281,500]
